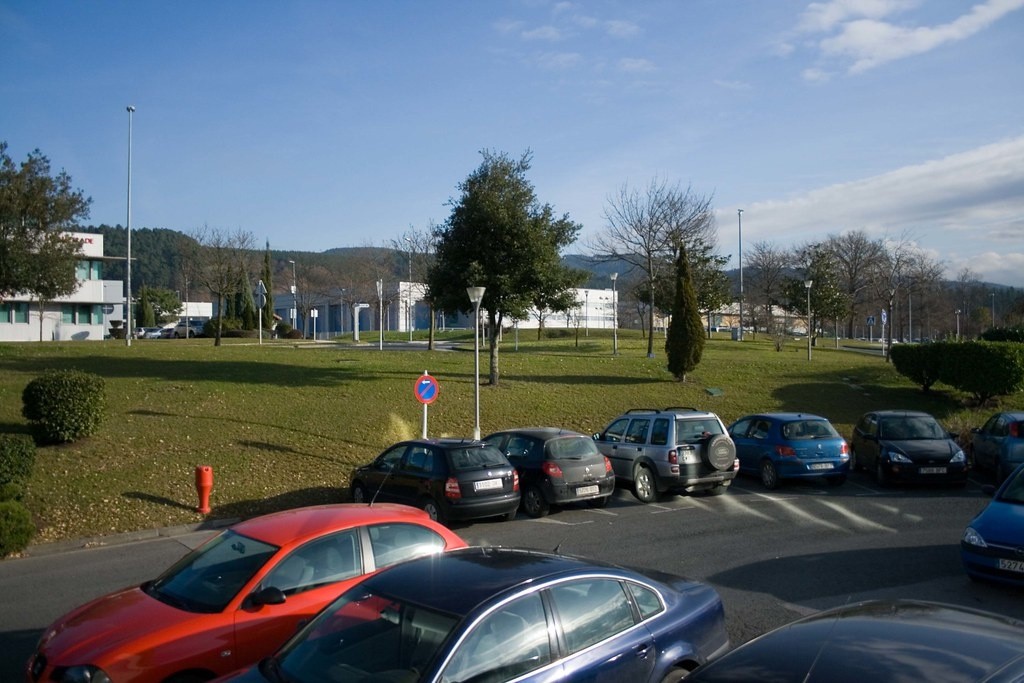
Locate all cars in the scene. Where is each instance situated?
[850,409,969,490]
[958,462,1023,597]
[229,545,731,683]
[673,597,1024,683]
[123,319,206,339]
[480,428,617,519]
[22,501,470,683]
[349,439,521,529]
[969,410,1024,485]
[725,413,851,492]
[840,336,930,344]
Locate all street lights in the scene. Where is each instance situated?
[404,238,413,341]
[609,271,618,353]
[465,287,486,444]
[803,279,813,362]
[289,260,298,329]
[584,290,590,336]
[955,309,962,335]
[126,105,136,347]
[737,208,744,341]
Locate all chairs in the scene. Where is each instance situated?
[468,611,534,683]
[751,422,768,439]
[411,453,433,472]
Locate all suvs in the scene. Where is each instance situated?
[591,406,740,503]
